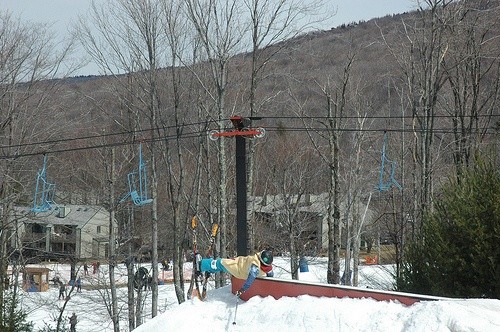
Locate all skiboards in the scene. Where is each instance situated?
[192,216,203,302]
[202,224,219,299]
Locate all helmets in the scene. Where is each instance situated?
[260,250,273,267]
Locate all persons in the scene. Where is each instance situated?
[76,276,82,292]
[144,275,149,292]
[83,262,90,277]
[58,282,65,300]
[195,250,274,297]
[92,260,100,274]
[68,312,77,332]
[148,275,153,292]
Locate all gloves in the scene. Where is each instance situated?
[236,288,245,297]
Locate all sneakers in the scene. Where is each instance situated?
[205,272,210,283]
[195,253,202,275]
